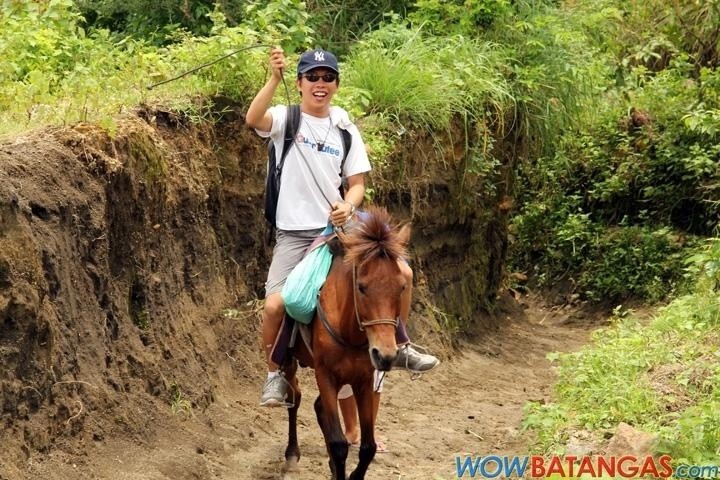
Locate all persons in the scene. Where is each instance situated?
[245,45,443,408]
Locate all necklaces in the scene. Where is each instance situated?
[301,112,332,152]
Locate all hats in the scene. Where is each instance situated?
[303,73,337,82]
[297,49,339,77]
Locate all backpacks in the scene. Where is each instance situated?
[281,227,334,325]
[264,139,277,228]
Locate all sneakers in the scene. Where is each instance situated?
[258,369,289,408]
[388,344,440,374]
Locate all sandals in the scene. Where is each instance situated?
[345,434,390,455]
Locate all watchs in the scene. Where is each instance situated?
[345,201,357,215]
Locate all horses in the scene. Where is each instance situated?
[284,204,414,480]
